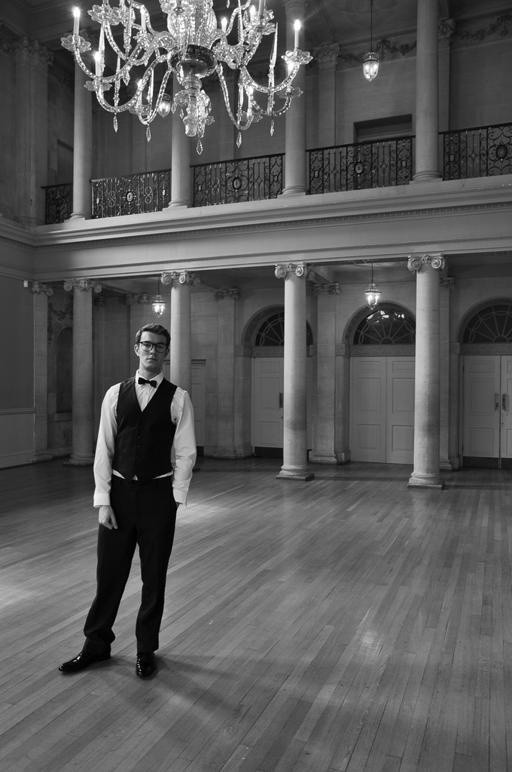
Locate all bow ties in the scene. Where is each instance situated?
[138,378,157,388]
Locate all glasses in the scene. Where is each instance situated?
[138,340,169,354]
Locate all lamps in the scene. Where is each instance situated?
[365,262,382,311]
[151,276,168,317]
[60,0,314,156]
[362,0,379,83]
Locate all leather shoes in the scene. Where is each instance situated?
[135,652,158,679]
[58,647,112,673]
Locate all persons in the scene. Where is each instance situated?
[58,322,198,679]
